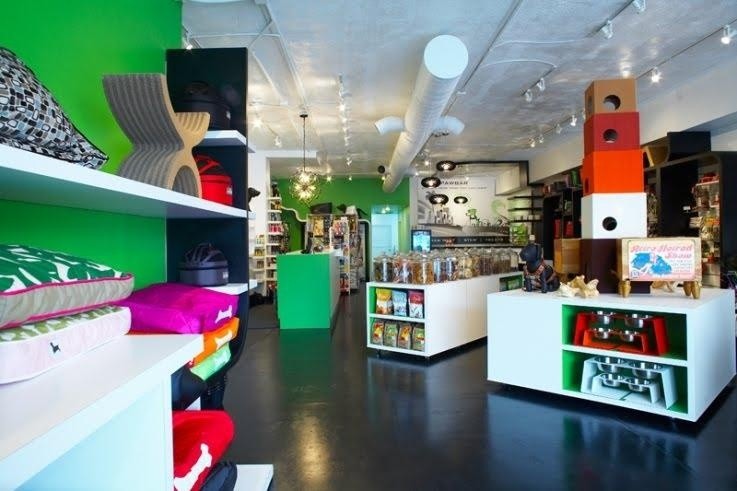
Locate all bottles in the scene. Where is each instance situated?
[393,253,413,284]
[458,251,473,279]
[499,250,511,274]
[509,250,519,271]
[432,251,448,282]
[480,251,493,275]
[470,250,481,277]
[447,250,459,281]
[412,252,435,284]
[492,250,502,274]
[373,252,394,282]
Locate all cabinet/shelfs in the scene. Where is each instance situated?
[486,281,736,433]
[0,47,274,491]
[304,212,359,296]
[276,247,340,331]
[542,150,737,289]
[366,272,524,367]
[248,196,291,298]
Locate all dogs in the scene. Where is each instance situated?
[520,243,561,293]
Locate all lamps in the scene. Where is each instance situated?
[288,114,323,208]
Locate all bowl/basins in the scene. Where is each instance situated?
[600,373,625,387]
[591,328,614,340]
[593,356,629,373]
[591,311,616,324]
[627,377,655,392]
[620,331,640,341]
[623,313,653,328]
[631,361,663,379]
